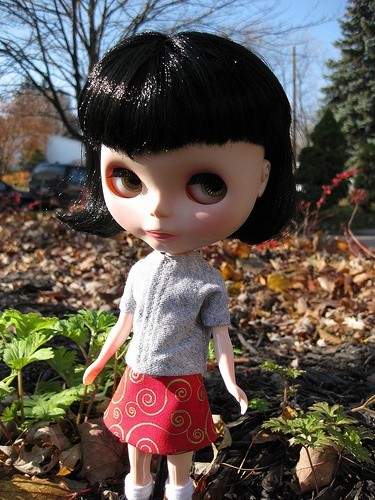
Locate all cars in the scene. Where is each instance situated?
[30,163,86,197]
